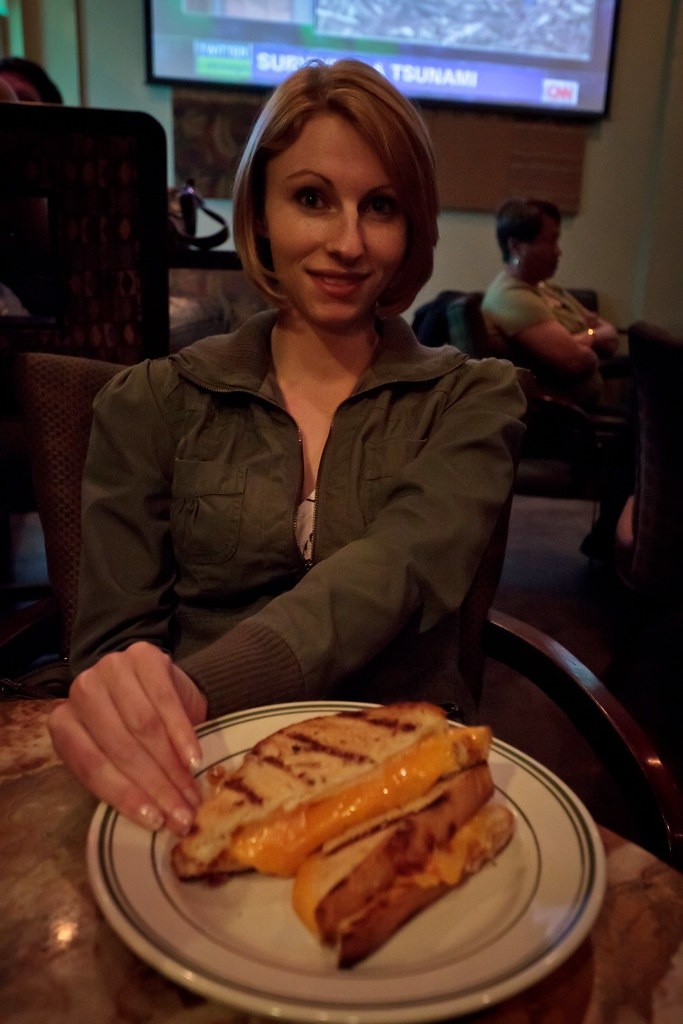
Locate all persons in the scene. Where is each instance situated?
[0,56,63,107]
[45,58,530,837]
[480,197,638,568]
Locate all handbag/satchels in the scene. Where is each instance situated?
[166,180,229,254]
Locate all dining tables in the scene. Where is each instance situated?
[0,697,683,1024]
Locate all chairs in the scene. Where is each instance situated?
[0,352,669,862]
[0,98,230,364]
[449,289,636,567]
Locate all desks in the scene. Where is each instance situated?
[167,198,242,271]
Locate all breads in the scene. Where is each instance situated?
[168,697,449,878]
[287,726,517,973]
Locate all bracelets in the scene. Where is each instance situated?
[587,327,598,342]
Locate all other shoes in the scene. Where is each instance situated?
[580,533,633,562]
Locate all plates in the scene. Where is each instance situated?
[86,701,607,1024]
